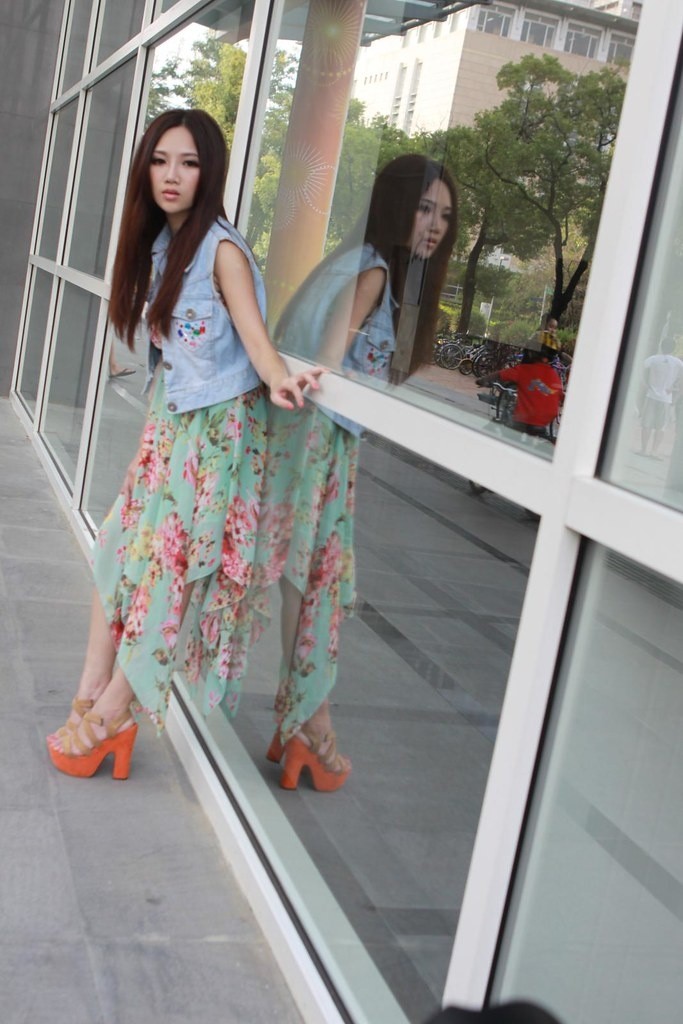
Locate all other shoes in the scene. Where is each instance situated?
[634,450,665,461]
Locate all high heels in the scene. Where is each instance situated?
[267,728,351,793]
[50,711,139,781]
[49,697,95,754]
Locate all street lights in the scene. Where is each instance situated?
[482,255,510,338]
[538,277,554,327]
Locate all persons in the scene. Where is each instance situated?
[46,105,329,780]
[262,154,457,789]
[522,316,559,354]
[475,343,562,436]
[632,336,683,460]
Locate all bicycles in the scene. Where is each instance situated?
[424,330,567,385]
[489,380,561,446]
[468,382,543,524]
